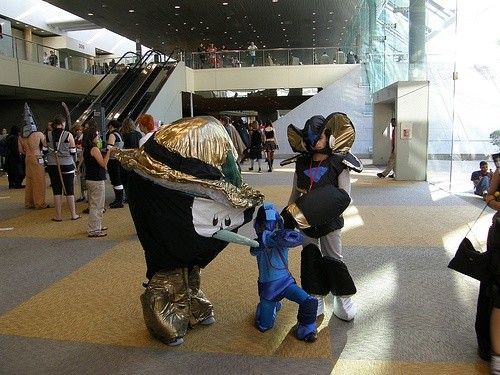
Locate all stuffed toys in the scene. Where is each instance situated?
[114,116,265,346]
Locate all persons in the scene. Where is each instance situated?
[250,203,318,341]
[334,48,343,60]
[92,62,117,74]
[0,117,142,213]
[229,56,239,67]
[247,41,258,67]
[216,114,266,173]
[264,118,279,172]
[475,168,500,375]
[137,113,156,148]
[377,118,395,178]
[322,52,328,56]
[471,161,494,200]
[278,112,363,322]
[45,119,82,220]
[102,120,124,208]
[198,43,228,68]
[44,51,58,66]
[81,127,116,237]
[18,131,53,209]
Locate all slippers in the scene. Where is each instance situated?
[52,218,62,222]
[72,215,82,220]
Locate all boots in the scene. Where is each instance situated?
[109,189,124,209]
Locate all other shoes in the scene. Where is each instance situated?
[102,227,108,231]
[492,354,500,375]
[305,333,317,342]
[377,173,385,178]
[88,233,107,237]
[389,174,394,179]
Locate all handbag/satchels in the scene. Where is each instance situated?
[447,240,487,281]
